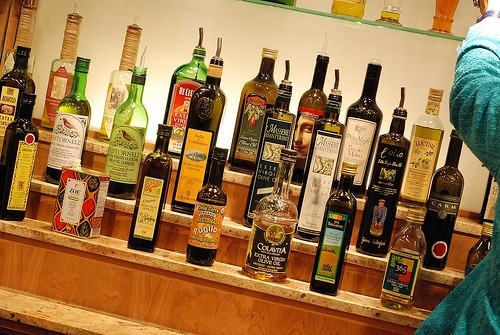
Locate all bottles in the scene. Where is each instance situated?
[0,90,39,221]
[328,60,387,197]
[103,65,150,198]
[128,122,176,252]
[241,147,300,284]
[155,27,209,158]
[356,88,412,256]
[172,37,226,216]
[40,13,85,130]
[226,48,280,172]
[2,0,40,79]
[0,46,38,162]
[293,69,346,239]
[400,88,447,204]
[288,55,330,185]
[308,160,358,295]
[480,174,500,224]
[99,24,145,143]
[465,222,494,280]
[242,58,296,229]
[45,56,92,185]
[421,128,465,272]
[260,0,463,35]
[186,148,228,266]
[379,207,429,311]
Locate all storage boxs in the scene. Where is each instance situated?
[50,166,110,237]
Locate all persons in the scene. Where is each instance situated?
[416,0,500,335]
[293,113,320,159]
